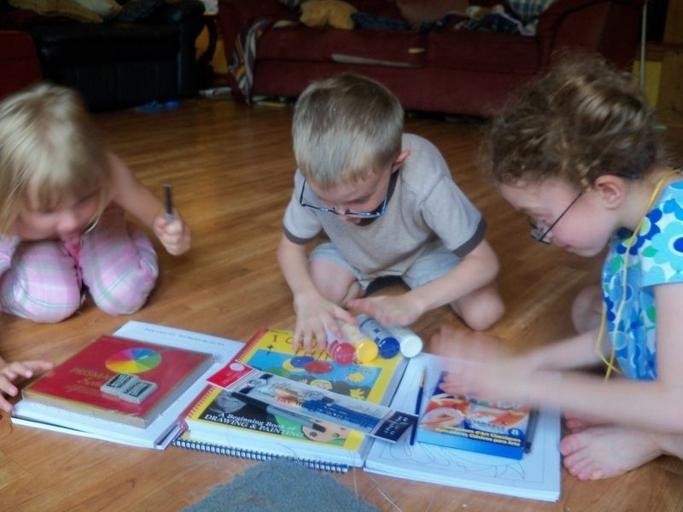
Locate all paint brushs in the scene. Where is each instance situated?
[410,369,429,446]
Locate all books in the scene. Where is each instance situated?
[9,317,563,501]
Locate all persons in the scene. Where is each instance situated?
[0,80,195,419]
[431,43,683,481]
[275,68,507,354]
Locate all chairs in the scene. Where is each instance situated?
[0,0,206,113]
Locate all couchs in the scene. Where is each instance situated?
[218,1,644,118]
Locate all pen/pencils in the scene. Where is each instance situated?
[166,190,172,220]
[273,386,379,429]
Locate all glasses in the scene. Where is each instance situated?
[298,178,389,220]
[528,186,587,247]
